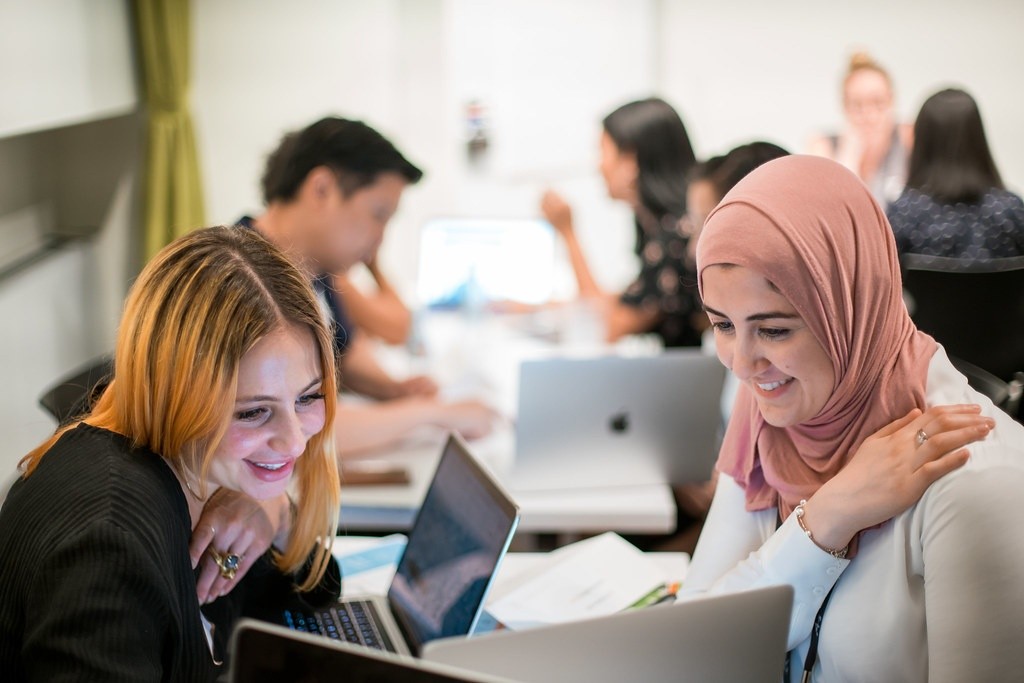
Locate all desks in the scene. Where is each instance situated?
[287,302,690,595]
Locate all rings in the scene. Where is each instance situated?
[209,546,239,581]
[915,430,929,444]
[199,522,216,535]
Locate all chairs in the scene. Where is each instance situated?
[903,253,1024,425]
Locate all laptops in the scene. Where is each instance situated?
[219,430,795,683]
[509,352,727,491]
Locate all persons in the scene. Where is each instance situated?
[229,117,509,460]
[540,52,1024,683]
[0,225,343,683]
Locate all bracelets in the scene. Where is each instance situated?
[796,499,848,559]
